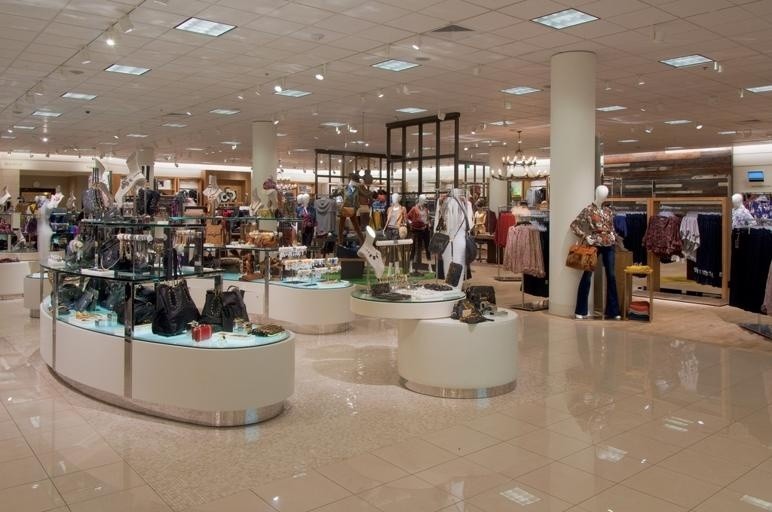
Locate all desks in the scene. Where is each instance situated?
[595,248,634,317]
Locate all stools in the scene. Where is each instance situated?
[621,269,654,323]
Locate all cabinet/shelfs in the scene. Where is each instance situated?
[153,176,203,209]
[0,209,306,283]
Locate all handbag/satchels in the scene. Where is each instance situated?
[59,277,154,325]
[343,207,355,217]
[466,239,477,264]
[451,286,496,324]
[358,205,370,214]
[371,284,411,301]
[97,239,120,270]
[206,224,225,245]
[566,245,598,271]
[245,231,276,247]
[428,233,450,255]
[198,286,249,332]
[446,263,462,287]
[248,323,284,337]
[152,280,201,337]
[190,249,279,281]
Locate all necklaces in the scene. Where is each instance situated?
[209,184,218,198]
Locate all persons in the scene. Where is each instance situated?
[201,174,223,209]
[731,193,772,229]
[0,151,147,273]
[571,185,622,321]
[252,173,547,289]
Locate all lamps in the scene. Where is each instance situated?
[489,128,550,182]
